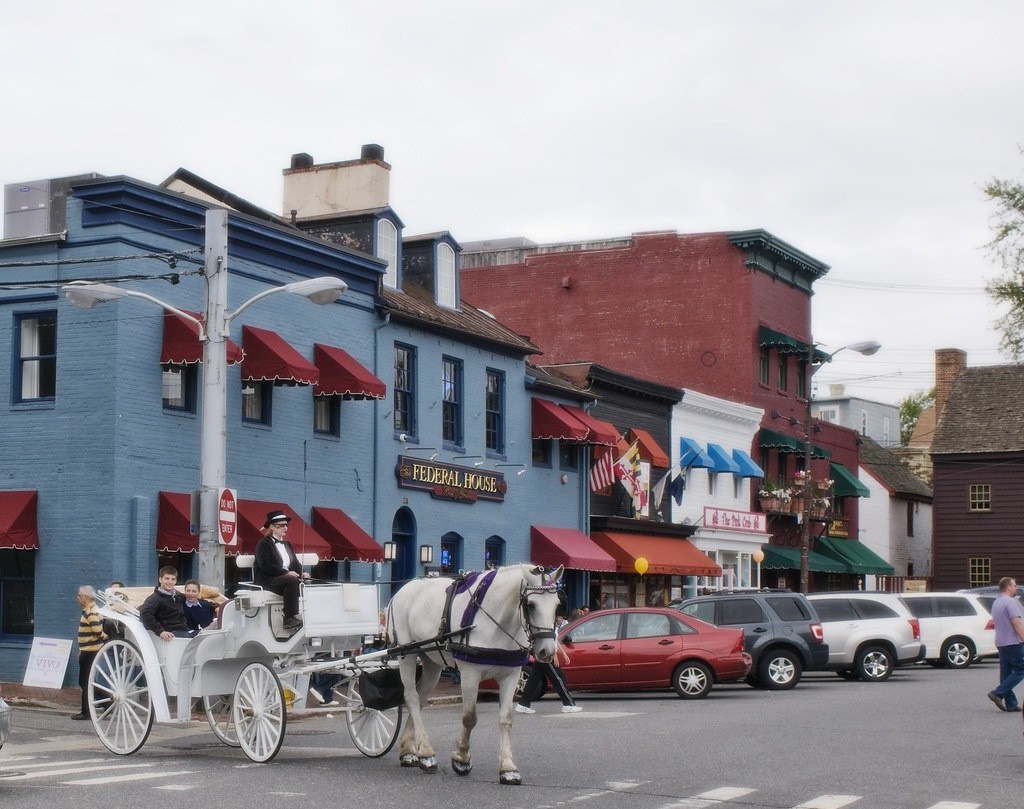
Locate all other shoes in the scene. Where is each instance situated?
[988,691,1007,711]
[310,685,325,702]
[319,701,340,706]
[562,705,582,712]
[283,615,302,628]
[71,713,91,719]
[1007,706,1022,711]
[514,704,535,713]
[103,700,115,718]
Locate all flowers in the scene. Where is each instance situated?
[757,469,835,509]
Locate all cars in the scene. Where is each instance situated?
[0,696,14,750]
[478,607,753,700]
[956,585,1024,607]
[804,590,927,682]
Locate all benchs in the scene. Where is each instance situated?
[136,599,235,636]
[233,553,318,606]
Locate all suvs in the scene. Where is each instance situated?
[675,587,831,690]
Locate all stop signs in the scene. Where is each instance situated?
[217,487,237,547]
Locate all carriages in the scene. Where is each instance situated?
[87,562,569,785]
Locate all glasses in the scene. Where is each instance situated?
[273,523,289,527]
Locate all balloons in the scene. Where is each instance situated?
[753,550,764,563]
[634,557,649,576]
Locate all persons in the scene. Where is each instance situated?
[70,566,220,720]
[515,607,590,714]
[310,651,340,706]
[987,576,1024,712]
[254,510,311,629]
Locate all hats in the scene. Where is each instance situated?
[264,509,292,528]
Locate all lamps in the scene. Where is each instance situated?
[453,455,485,467]
[791,416,800,425]
[404,447,439,461]
[814,424,823,432]
[420,544,433,564]
[496,463,527,475]
[772,410,782,419]
[383,541,397,561]
[656,511,664,523]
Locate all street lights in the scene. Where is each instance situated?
[61,208,350,599]
[800,334,882,596]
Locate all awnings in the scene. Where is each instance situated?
[529,524,723,578]
[629,427,669,468]
[680,437,715,469]
[531,398,589,441]
[156,491,384,564]
[593,420,630,461]
[242,326,320,389]
[707,443,740,474]
[313,342,386,400]
[732,449,765,479]
[752,537,895,576]
[160,307,247,367]
[0,490,40,550]
[559,404,617,447]
[759,427,870,499]
[759,327,833,364]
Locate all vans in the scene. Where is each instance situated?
[899,592,1000,668]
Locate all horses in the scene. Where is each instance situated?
[385,563,564,785]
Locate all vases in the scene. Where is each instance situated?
[818,482,829,490]
[794,479,805,486]
[759,496,826,516]
